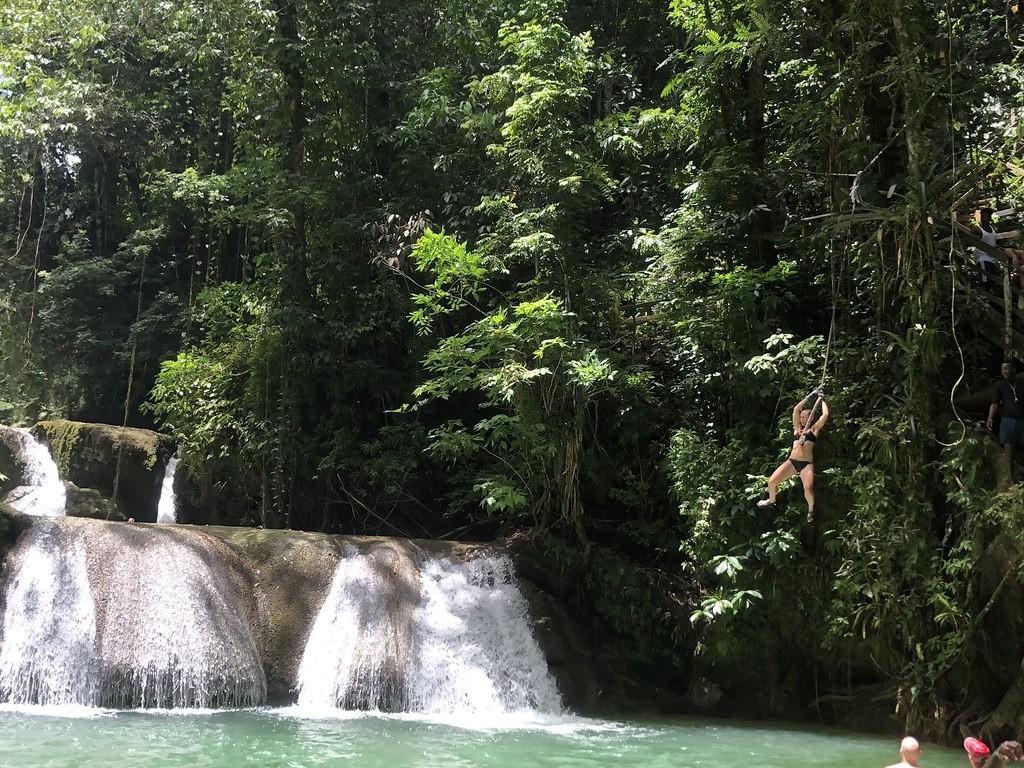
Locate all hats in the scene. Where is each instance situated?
[964,737,990,758]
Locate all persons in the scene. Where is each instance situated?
[757,385,828,524]
[885,736,921,768]
[964,737,1024,768]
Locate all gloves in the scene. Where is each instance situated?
[806,383,827,406]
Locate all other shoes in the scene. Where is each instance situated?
[758,498,777,509]
[807,511,814,523]
